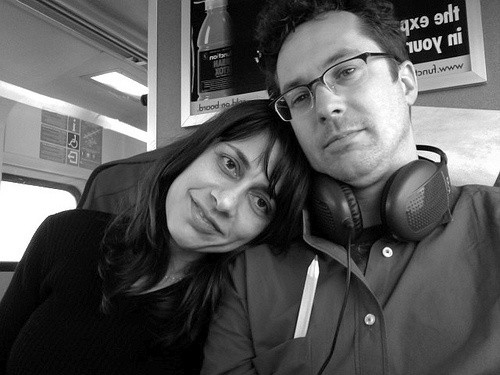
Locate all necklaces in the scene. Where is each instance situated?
[164,274,182,282]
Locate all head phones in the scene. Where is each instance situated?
[306,145,451,246]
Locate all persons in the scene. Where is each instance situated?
[0,99,321,375]
[202,0,500,375]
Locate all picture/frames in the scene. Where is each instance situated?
[394,0,487,95]
[180,0,271,128]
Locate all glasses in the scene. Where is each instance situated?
[268,51,401,122]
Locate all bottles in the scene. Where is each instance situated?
[196,0,235,100]
